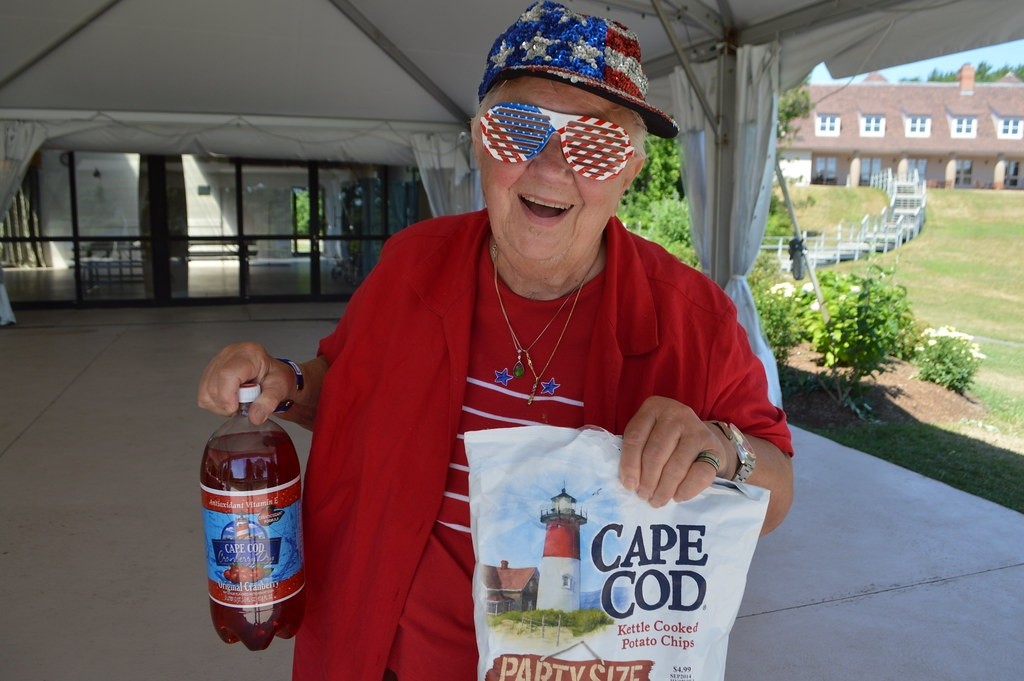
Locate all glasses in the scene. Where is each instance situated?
[479,102,644,182]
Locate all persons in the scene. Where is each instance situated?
[196,0,794,681]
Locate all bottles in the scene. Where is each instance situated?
[200,382,305,651]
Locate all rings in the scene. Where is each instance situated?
[695,451,719,472]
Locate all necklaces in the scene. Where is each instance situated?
[491,242,601,405]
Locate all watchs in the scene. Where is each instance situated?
[703,421,756,483]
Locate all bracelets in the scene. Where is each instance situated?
[273,358,304,414]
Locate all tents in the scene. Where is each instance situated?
[0,0,1024,415]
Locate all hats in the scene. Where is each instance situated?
[478,1,679,139]
